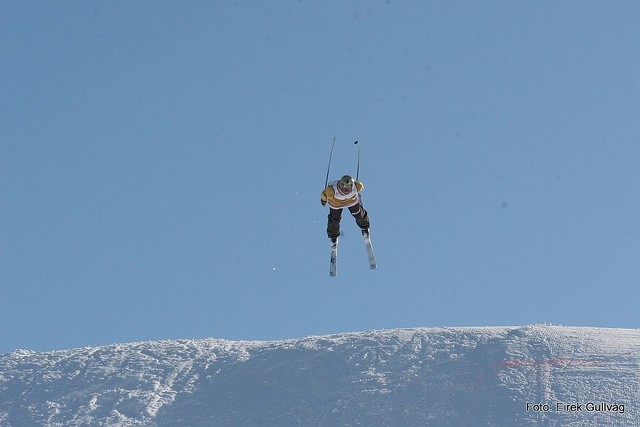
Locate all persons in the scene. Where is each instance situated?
[320,174,371,246]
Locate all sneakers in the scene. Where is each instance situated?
[361,229,370,238]
[331,235,337,246]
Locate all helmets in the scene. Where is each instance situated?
[340,175,354,190]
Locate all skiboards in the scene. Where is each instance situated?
[328,242,377,277]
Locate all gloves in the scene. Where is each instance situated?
[321,199,327,205]
